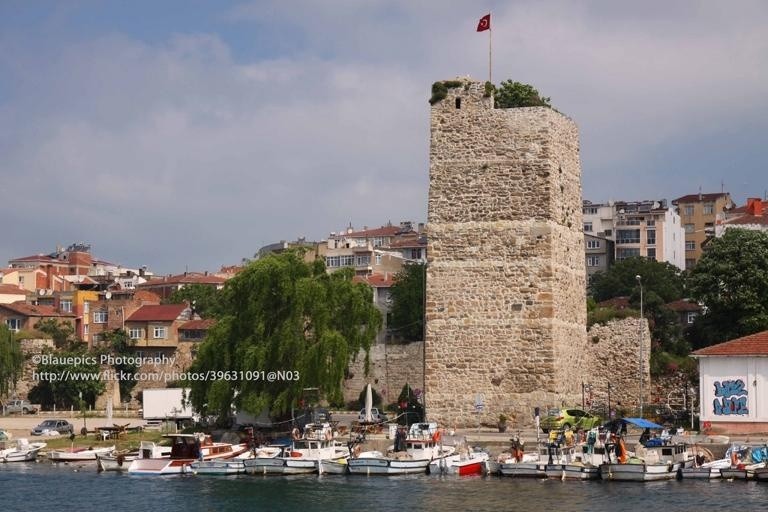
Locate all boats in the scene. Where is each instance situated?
[0,426,768,480]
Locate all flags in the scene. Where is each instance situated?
[476,13,490,32]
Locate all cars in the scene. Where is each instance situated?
[29,419,73,437]
[538,406,602,434]
[316,409,332,425]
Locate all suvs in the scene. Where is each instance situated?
[357,406,389,425]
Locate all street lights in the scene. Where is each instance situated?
[634,274,643,420]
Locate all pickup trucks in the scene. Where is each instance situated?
[1,399,42,416]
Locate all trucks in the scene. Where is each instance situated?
[141,385,242,431]
